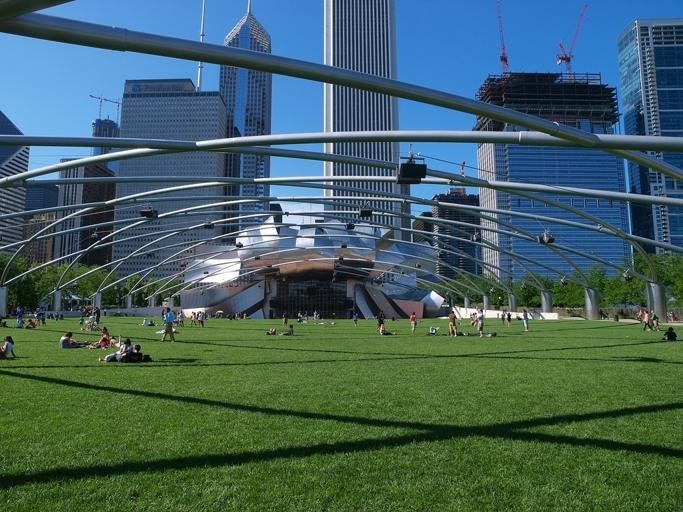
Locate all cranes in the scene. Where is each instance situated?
[495,0,507,75]
[90,93,123,128]
[556,3,587,80]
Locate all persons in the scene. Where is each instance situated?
[352,310,358,327]
[282,310,288,327]
[159,307,177,343]
[376,309,386,336]
[265,328,275,336]
[146,320,154,326]
[160,309,165,325]
[296,309,320,325]
[637,307,660,333]
[210,311,249,321]
[69,326,144,365]
[13,304,63,330]
[78,305,101,331]
[466,331,496,338]
[599,309,603,320]
[0,335,16,358]
[0,318,8,327]
[506,312,511,327]
[469,312,477,327]
[521,308,528,332]
[276,323,294,336]
[662,326,677,341]
[409,311,416,334]
[501,311,505,324]
[474,308,484,338]
[58,331,89,348]
[448,311,457,338]
[140,317,147,327]
[171,310,211,329]
